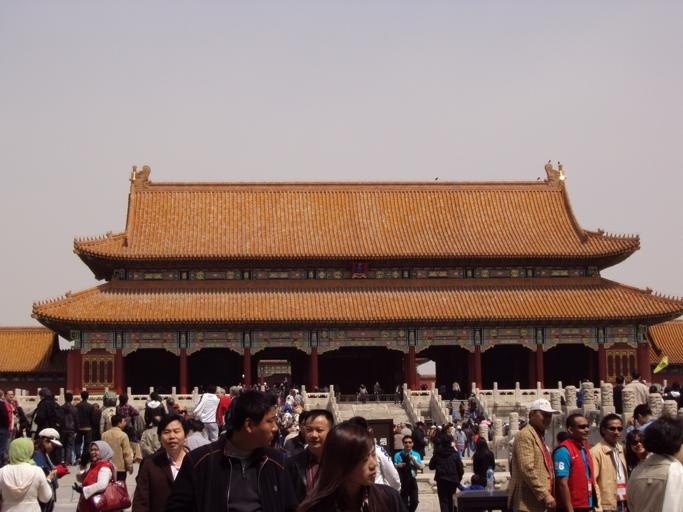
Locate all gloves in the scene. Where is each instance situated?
[72,483,83,494]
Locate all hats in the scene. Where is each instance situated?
[529,398,560,415]
[39,427,63,447]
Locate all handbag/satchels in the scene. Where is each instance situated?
[90,463,132,512]
[133,416,145,434]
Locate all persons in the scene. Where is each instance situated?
[0,367,683,512]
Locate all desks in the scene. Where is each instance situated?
[452,492,510,512]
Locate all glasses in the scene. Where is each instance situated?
[608,426,623,432]
[403,442,412,444]
[576,423,589,429]
[630,439,642,446]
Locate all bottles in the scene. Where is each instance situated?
[486,467,494,493]
